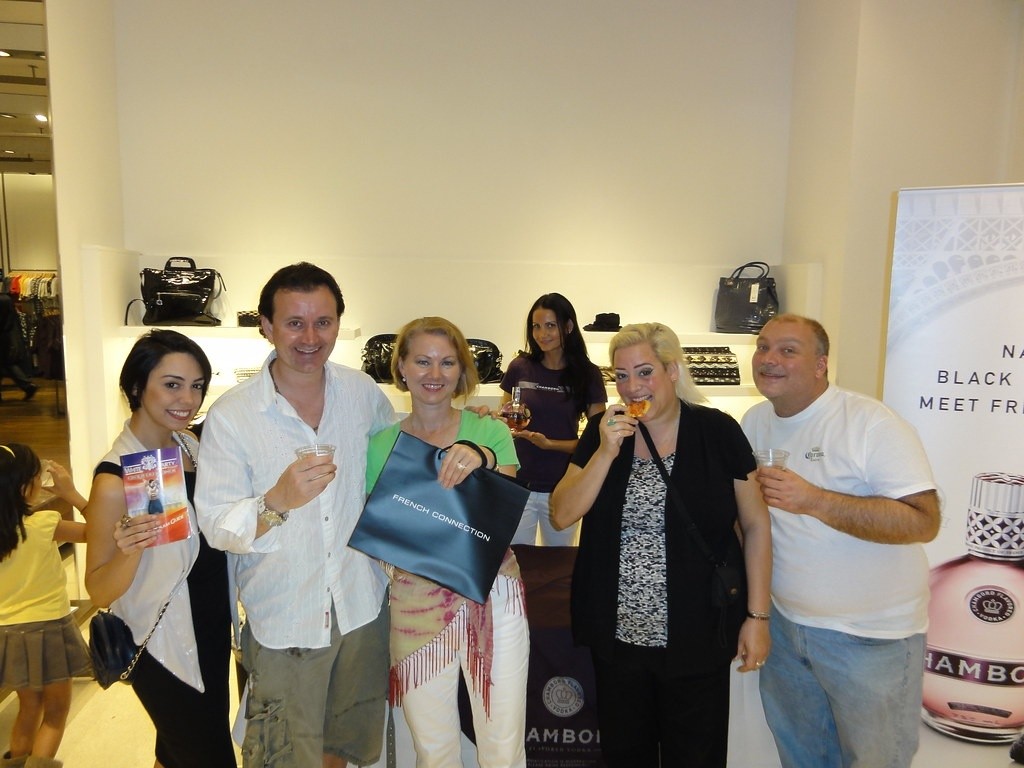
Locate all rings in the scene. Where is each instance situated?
[756,660,765,667]
[457,463,466,470]
[617,431,621,438]
[120,514,131,528]
[607,416,616,426]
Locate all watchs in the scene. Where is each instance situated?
[258,494,289,527]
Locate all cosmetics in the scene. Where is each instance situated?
[919,472,1024,744]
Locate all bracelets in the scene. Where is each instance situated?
[748,610,770,620]
[80,505,87,515]
[483,445,498,470]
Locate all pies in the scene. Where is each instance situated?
[623,400,650,417]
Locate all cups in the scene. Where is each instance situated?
[752,448,791,478]
[294,445,337,479]
[41,459,56,488]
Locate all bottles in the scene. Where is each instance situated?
[920,472,1024,743]
[498,386,532,432]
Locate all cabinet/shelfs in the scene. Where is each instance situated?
[119,326,762,414]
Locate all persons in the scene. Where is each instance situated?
[550,322,772,768]
[193,262,509,768]
[366,316,530,768]
[499,293,608,546]
[0,444,88,768]
[84,330,238,768]
[0,294,38,401]
[739,314,942,768]
[146,481,164,515]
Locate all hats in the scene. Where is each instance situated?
[583,312,624,332]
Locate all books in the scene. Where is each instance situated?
[120,447,191,547]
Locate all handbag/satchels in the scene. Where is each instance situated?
[348,430,533,606]
[360,334,402,383]
[466,339,504,383]
[88,612,139,690]
[710,566,747,608]
[140,257,221,326]
[715,262,779,334]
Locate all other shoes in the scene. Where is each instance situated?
[24,384,39,400]
[25,757,62,768]
[1,750,30,768]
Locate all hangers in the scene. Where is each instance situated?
[41,308,61,317]
[8,269,56,279]
